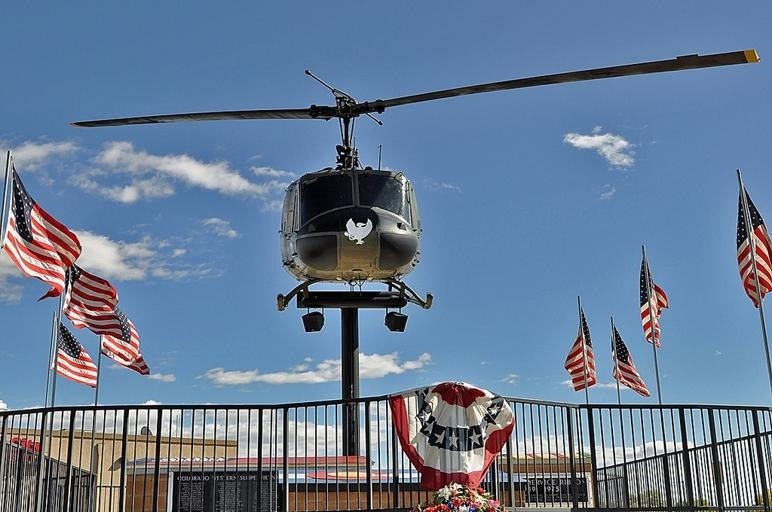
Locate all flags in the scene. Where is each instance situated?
[636,257,667,347]
[50,322,99,389]
[64,262,132,344]
[561,307,598,391]
[100,312,151,379]
[734,187,771,311]
[5,169,83,304]
[609,323,650,399]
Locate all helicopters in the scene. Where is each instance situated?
[69,48,763,333]
